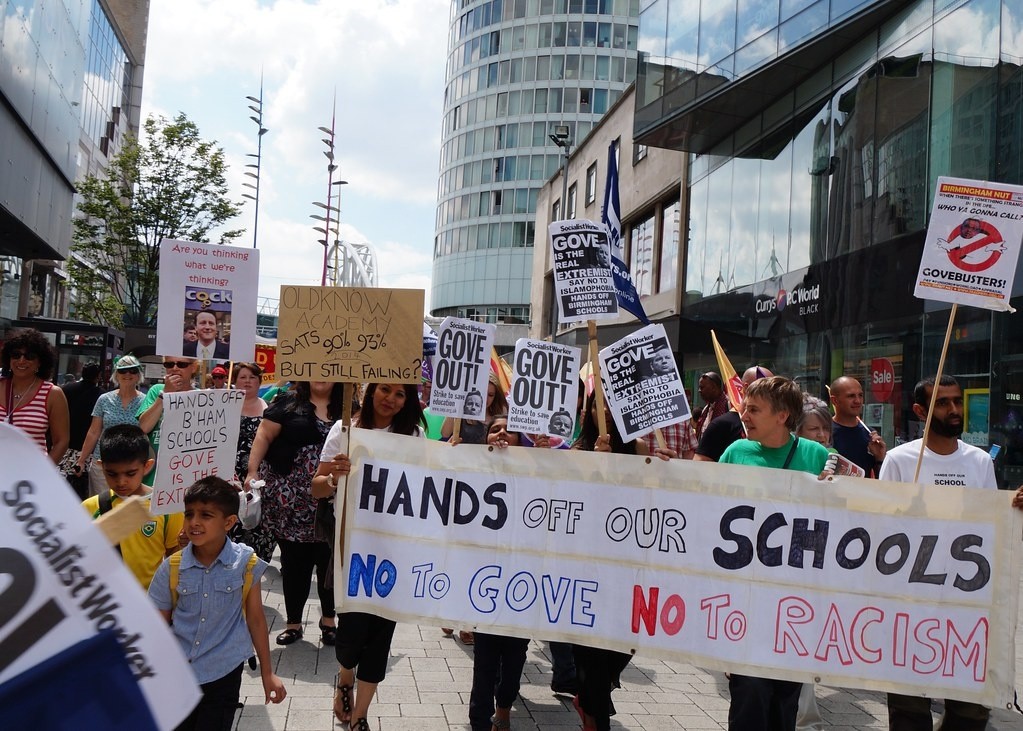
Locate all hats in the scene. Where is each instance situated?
[212,366,226,375]
[116,352,140,369]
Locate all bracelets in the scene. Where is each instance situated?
[158,394,163,400]
[160,390,165,396]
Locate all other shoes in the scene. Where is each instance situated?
[491,712,510,731]
[276,624,303,645]
[460,630,473,645]
[319,616,336,645]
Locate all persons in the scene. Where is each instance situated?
[0,325,433,644]
[463,391,483,416]
[312,385,428,731]
[695,366,885,731]
[650,344,675,380]
[183,309,230,359]
[548,410,573,438]
[421,374,699,731]
[595,240,610,269]
[873,378,1023,731]
[148,475,286,731]
[712,376,842,731]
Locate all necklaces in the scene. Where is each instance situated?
[12,375,35,399]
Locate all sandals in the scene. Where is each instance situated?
[334,670,355,724]
[348,713,370,731]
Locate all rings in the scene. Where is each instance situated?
[177,382,181,386]
[336,465,340,470]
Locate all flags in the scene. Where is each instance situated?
[711,329,746,412]
[490,345,514,397]
[0,626,161,731]
[602,140,651,325]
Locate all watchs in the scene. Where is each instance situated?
[326,473,338,488]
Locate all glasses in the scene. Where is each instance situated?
[116,367,139,374]
[162,361,194,368]
[11,349,36,361]
[803,401,828,410]
[212,375,225,380]
[698,374,718,386]
[251,363,259,368]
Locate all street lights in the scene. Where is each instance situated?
[548,125,575,350]
[810,155,840,263]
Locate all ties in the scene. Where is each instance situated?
[202,346,208,358]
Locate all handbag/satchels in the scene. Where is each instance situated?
[238,478,265,530]
